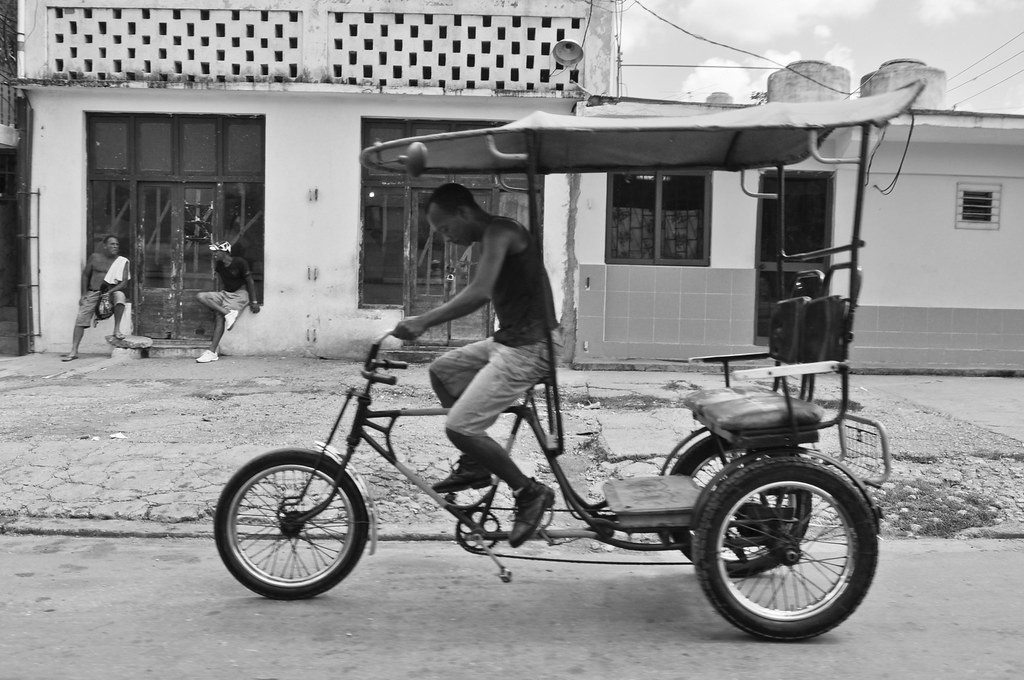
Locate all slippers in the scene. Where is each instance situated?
[61,355,78,361]
[113,333,125,340]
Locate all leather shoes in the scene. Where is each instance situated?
[432,455,493,493]
[509,477,555,548]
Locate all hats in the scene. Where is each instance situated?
[208,241,232,253]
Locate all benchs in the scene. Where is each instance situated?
[686,261,863,448]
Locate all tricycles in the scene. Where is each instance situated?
[211,73,928,642]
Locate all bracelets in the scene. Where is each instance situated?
[252,301,258,304]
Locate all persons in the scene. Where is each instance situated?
[392,183,559,548]
[63,236,128,361]
[196,240,260,363]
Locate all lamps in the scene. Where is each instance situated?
[552,38,584,66]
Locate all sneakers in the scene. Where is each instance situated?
[195,349,219,363]
[224,310,239,330]
